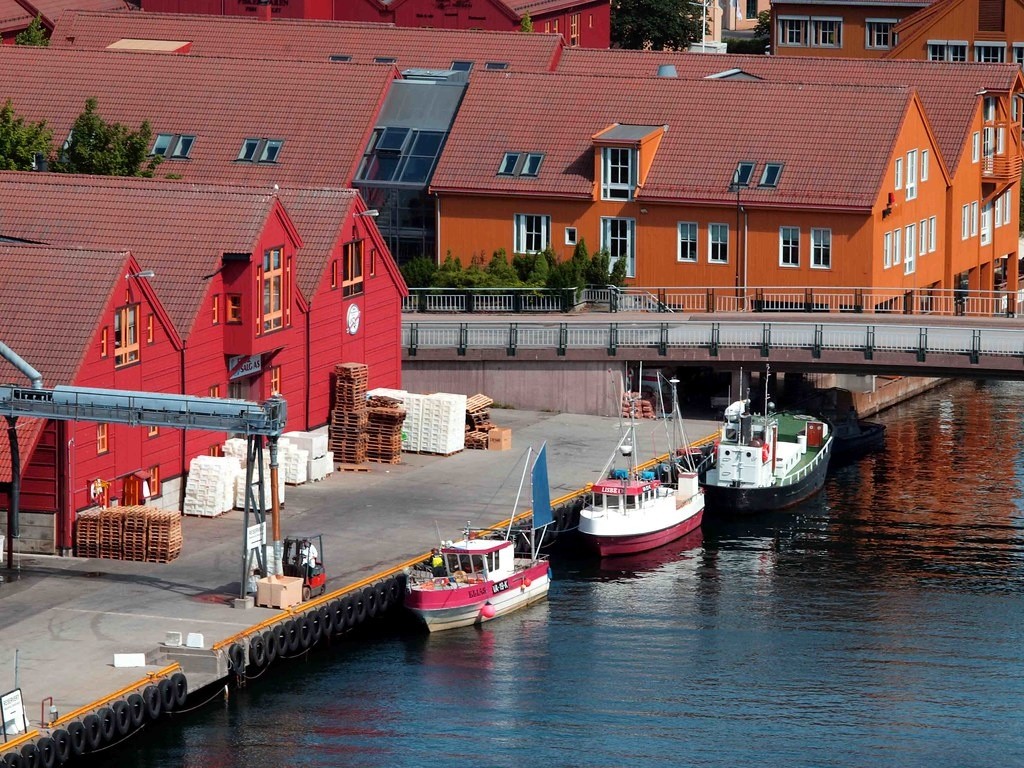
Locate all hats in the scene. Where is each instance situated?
[302,537,311,543]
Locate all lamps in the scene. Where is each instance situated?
[353,210,379,218]
[125,271,154,280]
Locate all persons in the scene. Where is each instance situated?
[430,547,446,576]
[299,540,318,578]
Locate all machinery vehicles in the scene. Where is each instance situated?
[279,532,326,601]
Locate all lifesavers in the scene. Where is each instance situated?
[466,578,482,583]
[762,444,770,461]
[712,441,720,457]
[435,577,448,588]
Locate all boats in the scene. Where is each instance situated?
[573,359,708,557]
[700,367,836,516]
[405,446,553,632]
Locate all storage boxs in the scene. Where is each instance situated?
[256,575,304,609]
[308,452,334,481]
[280,431,327,460]
[487,427,512,451]
